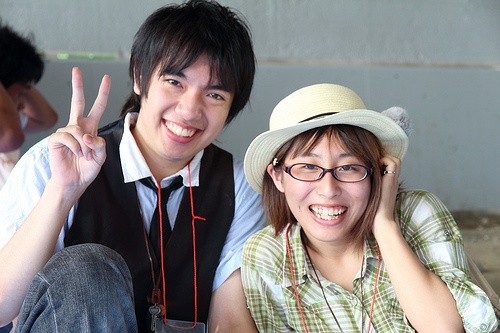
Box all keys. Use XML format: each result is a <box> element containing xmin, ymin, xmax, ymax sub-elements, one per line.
<box><xmin>149</xmin><ymin>303</ymin><xmax>161</xmax><ymax>331</ymax></box>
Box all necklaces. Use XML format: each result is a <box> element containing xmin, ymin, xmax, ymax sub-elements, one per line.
<box><xmin>287</xmin><ymin>223</ymin><xmax>381</xmax><ymax>333</ymax></box>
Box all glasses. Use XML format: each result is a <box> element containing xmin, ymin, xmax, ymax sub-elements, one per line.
<box><xmin>280</xmin><ymin>164</ymin><xmax>374</xmax><ymax>183</ymax></box>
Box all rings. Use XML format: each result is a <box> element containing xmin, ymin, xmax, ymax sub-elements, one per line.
<box><xmin>383</xmin><ymin>171</ymin><xmax>397</xmax><ymax>174</ymax></box>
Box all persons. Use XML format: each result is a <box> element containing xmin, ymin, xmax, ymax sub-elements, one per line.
<box><xmin>241</xmin><ymin>83</ymin><xmax>500</xmax><ymax>333</ymax></box>
<box><xmin>0</xmin><ymin>26</ymin><xmax>57</xmax><ymax>191</ymax></box>
<box><xmin>0</xmin><ymin>0</ymin><xmax>267</xmax><ymax>332</ymax></box>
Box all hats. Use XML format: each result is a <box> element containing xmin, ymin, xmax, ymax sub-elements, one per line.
<box><xmin>243</xmin><ymin>83</ymin><xmax>409</xmax><ymax>194</ymax></box>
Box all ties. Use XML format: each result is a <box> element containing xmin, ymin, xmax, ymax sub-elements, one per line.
<box><xmin>136</xmin><ymin>176</ymin><xmax>184</xmax><ymax>265</ymax></box>
